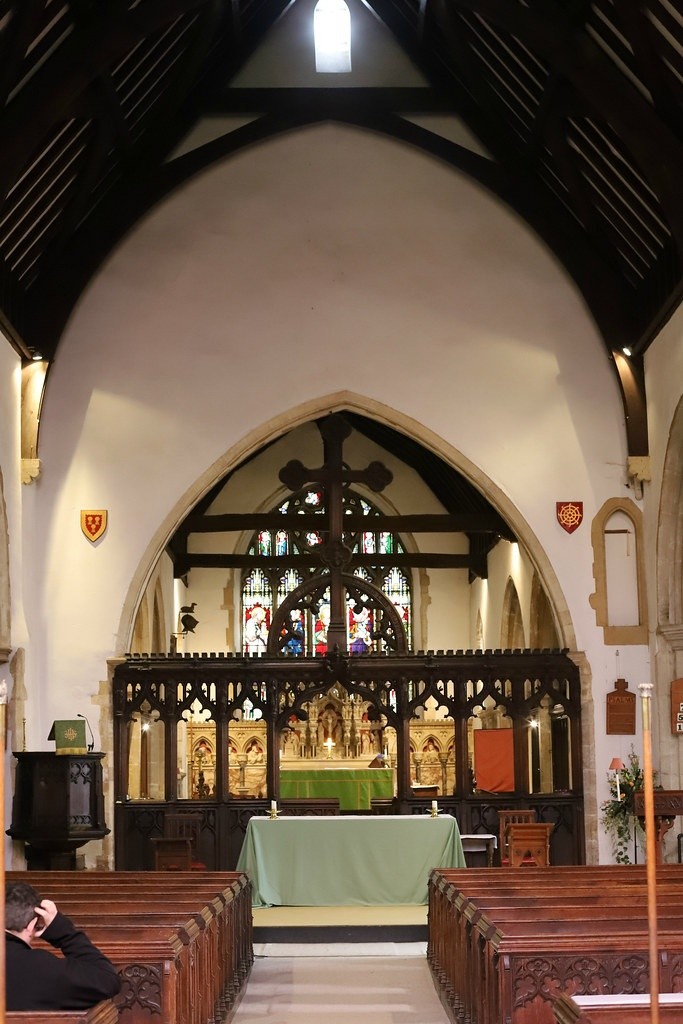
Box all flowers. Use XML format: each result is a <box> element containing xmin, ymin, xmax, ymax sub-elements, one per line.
<box><xmin>599</xmin><ymin>743</ymin><xmax>666</xmax><ymax>865</ymax></box>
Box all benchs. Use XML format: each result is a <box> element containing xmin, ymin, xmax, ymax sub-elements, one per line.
<box><xmin>427</xmin><ymin>865</ymin><xmax>683</xmax><ymax>1024</ymax></box>
<box><xmin>4</xmin><ymin>870</ymin><xmax>255</xmax><ymax>1024</ymax></box>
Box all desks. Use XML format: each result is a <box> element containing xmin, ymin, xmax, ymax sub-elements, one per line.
<box><xmin>235</xmin><ymin>814</ymin><xmax>467</xmax><ymax>908</ymax></box>
<box><xmin>279</xmin><ymin>768</ymin><xmax>394</xmax><ymax>810</ymax></box>
<box><xmin>460</xmin><ymin>834</ymin><xmax>497</xmax><ymax>867</ymax></box>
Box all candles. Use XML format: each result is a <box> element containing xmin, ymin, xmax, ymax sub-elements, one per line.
<box><xmin>432</xmin><ymin>801</ymin><xmax>437</xmax><ymax>807</ymax></box>
<box><xmin>271</xmin><ymin>800</ymin><xmax>276</xmax><ymax>809</ymax></box>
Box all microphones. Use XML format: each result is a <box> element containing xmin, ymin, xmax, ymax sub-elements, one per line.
<box><xmin>77</xmin><ymin>714</ymin><xmax>94</xmax><ymax>751</ymax></box>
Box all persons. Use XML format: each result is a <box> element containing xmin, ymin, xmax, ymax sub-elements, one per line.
<box><xmin>335</xmin><ymin>723</ymin><xmax>342</xmax><ymax>743</ymax></box>
<box><xmin>362</xmin><ymin>731</ymin><xmax>370</xmax><ymax>754</ymax></box>
<box><xmin>5</xmin><ymin>880</ymin><xmax>122</xmax><ymax>1011</ymax></box>
<box><xmin>318</xmin><ymin>723</ymin><xmax>324</xmax><ymax>746</ymax></box>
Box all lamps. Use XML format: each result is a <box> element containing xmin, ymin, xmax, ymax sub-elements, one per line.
<box><xmin>610</xmin><ymin>758</ymin><xmax>625</xmax><ymax>801</ymax></box>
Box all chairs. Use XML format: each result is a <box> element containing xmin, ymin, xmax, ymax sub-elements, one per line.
<box><xmin>496</xmin><ymin>810</ymin><xmax>538</xmax><ymax>867</ymax></box>
